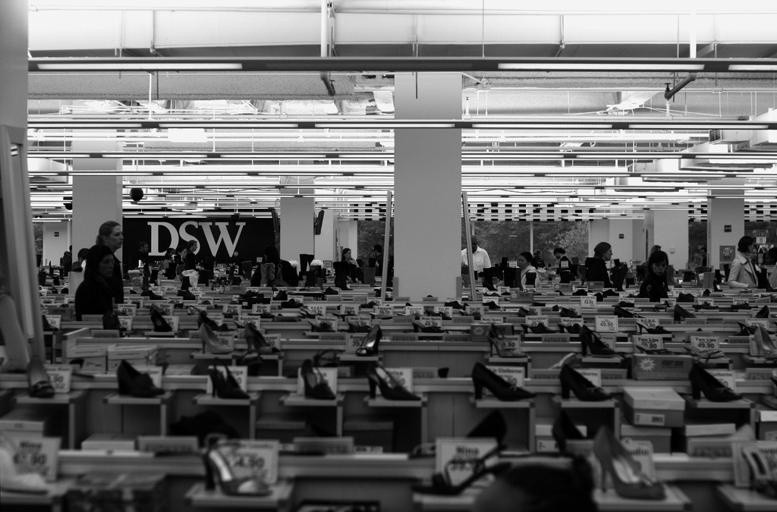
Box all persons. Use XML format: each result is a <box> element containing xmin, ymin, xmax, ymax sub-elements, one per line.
<box><xmin>135</xmin><ymin>234</ymin><xmax>396</xmax><ymax>291</ymax></box>
<box><xmin>582</xmin><ymin>241</ymin><xmax>674</xmax><ymax>303</ymax></box>
<box><xmin>728</xmin><ymin>232</ymin><xmax>776</xmax><ymax>294</ymax></box>
<box><xmin>74</xmin><ymin>221</ymin><xmax>126</xmax><ymax>330</ymax></box>
<box><xmin>71</xmin><ymin>247</ymin><xmax>90</xmax><ymax>271</ymax></box>
<box><xmin>459</xmin><ymin>233</ymin><xmax>579</xmax><ymax>289</ymax></box>
<box><xmin>62</xmin><ymin>245</ymin><xmax>72</xmax><ymax>277</ymax></box>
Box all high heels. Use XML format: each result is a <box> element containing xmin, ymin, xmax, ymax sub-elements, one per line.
<box><xmin>299</xmin><ymin>357</ymin><xmax>337</xmax><ymax>400</ymax></box>
<box><xmin>117</xmin><ymin>360</ymin><xmax>166</xmax><ymax>398</ymax></box>
<box><xmin>741</xmin><ymin>441</ymin><xmax>777</xmax><ymax>500</ymax></box>
<box><xmin>409</xmin><ymin>447</ymin><xmax>513</xmax><ymax>497</ymax></box>
<box><xmin>199</xmin><ymin>433</ymin><xmax>273</xmax><ymax>497</ymax></box>
<box><xmin>33</xmin><ymin>285</ymin><xmax>776</xmax><ymax>359</ymax></box>
<box><xmin>559</xmin><ymin>362</ymin><xmax>615</xmax><ymax>403</ymax></box>
<box><xmin>366</xmin><ymin>361</ymin><xmax>422</xmax><ymax>403</ymax></box>
<box><xmin>207</xmin><ymin>357</ymin><xmax>252</xmax><ymax>401</ymax></box>
<box><xmin>470</xmin><ymin>360</ymin><xmax>537</xmax><ymax>401</ymax></box>
<box><xmin>593</xmin><ymin>426</ymin><xmax>666</xmax><ymax>500</ymax></box>
<box><xmin>688</xmin><ymin>360</ymin><xmax>743</xmax><ymax>403</ymax></box>
<box><xmin>0</xmin><ymin>429</ymin><xmax>52</xmax><ymax>494</ymax></box>
<box><xmin>25</xmin><ymin>355</ymin><xmax>56</xmax><ymax>398</ymax></box>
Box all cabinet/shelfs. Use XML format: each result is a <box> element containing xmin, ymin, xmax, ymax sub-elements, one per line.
<box><xmin>0</xmin><ymin>257</ymin><xmax>777</xmax><ymax>512</ymax></box>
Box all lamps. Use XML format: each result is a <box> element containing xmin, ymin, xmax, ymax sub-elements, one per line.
<box><xmin>12</xmin><ymin>47</ymin><xmax>777</xmax><ymax>222</ymax></box>
